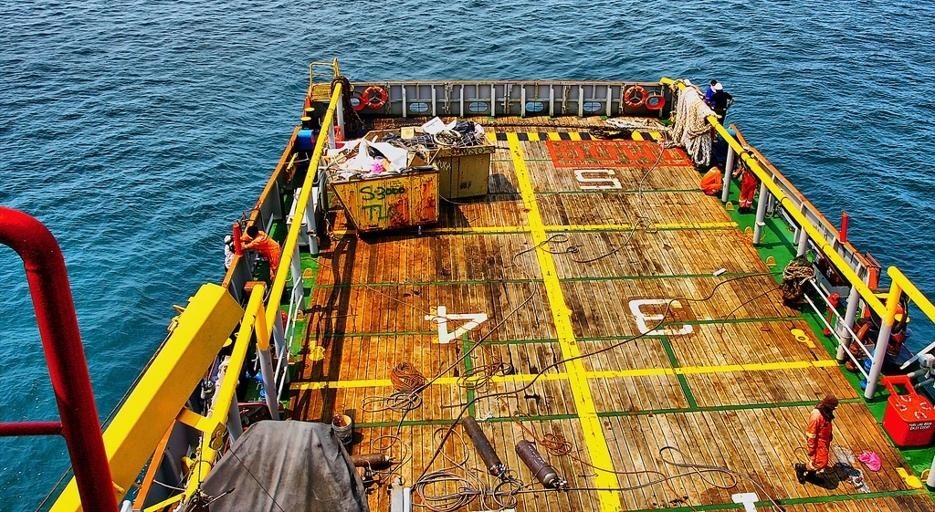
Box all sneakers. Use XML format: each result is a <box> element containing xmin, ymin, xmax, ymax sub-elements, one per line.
<box><xmin>794</xmin><ymin>463</ymin><xmax>816</xmax><ymax>485</ymax></box>
<box><xmin>738</xmin><ymin>207</ymin><xmax>751</xmax><ymax>213</ymax></box>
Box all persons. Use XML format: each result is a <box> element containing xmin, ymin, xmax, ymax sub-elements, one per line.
<box><xmin>707</xmin><ymin>82</ymin><xmax>735</xmax><ymax>128</ymax></box>
<box><xmin>737</xmin><ymin>165</ymin><xmax>760</xmax><ymax>213</ymax></box>
<box><xmin>223</xmin><ymin>234</ymin><xmax>236</xmax><ymax>273</ymax></box>
<box><xmin>704</xmin><ymin>80</ymin><xmax>718</xmax><ymax>110</ymax></box>
<box><xmin>238</xmin><ymin>225</ymin><xmax>283</xmax><ymax>279</ymax></box>
<box><xmin>699</xmin><ymin>161</ymin><xmax>725</xmax><ymax>196</ymax></box>
<box><xmin>794</xmin><ymin>394</ymin><xmax>838</xmax><ymax>484</ymax></box>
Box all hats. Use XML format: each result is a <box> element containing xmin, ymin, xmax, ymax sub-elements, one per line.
<box><xmin>713</xmin><ymin>83</ymin><xmax>723</xmax><ymax>90</ymax></box>
<box><xmin>823</xmin><ymin>394</ymin><xmax>838</xmax><ymax>409</ymax></box>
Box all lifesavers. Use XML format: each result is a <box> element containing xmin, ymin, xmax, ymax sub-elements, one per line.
<box><xmin>362</xmin><ymin>86</ymin><xmax>388</xmax><ymax>109</ymax></box>
<box><xmin>624</xmin><ymin>86</ymin><xmax>647</xmax><ymax>108</ymax></box>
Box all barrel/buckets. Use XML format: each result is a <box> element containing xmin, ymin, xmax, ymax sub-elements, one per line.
<box><xmin>331</xmin><ymin>415</ymin><xmax>353</xmax><ymax>445</ymax></box>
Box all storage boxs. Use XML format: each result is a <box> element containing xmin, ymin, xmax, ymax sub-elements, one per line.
<box><xmin>835</xmin><ymin>317</ymin><xmax>874</xmax><ymax>353</ymax></box>
<box><xmin>813</xmin><ymin>263</ymin><xmax>848</xmax><ymax>297</ymax></box>
<box><xmin>883</xmin><ymin>395</ymin><xmax>935</xmax><ymax>447</ymax></box>
<box><xmin>286</xmin><ymin>186</ymin><xmax>319</xmax><ymax>246</ymax></box>
